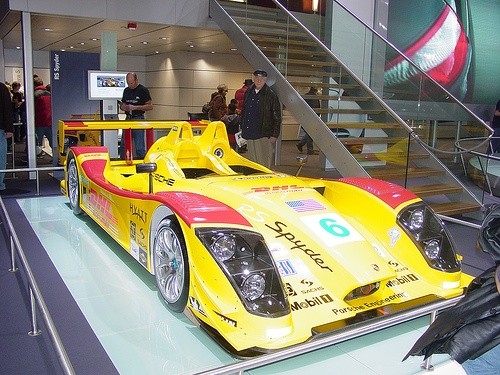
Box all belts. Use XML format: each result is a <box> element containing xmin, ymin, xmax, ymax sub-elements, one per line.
<box><xmin>127</xmin><ymin>115</ymin><xmax>142</xmax><ymax>118</ymax></box>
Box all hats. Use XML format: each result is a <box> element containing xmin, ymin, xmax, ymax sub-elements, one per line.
<box><xmin>217</xmin><ymin>83</ymin><xmax>228</xmax><ymax>90</ymax></box>
<box><xmin>243</xmin><ymin>79</ymin><xmax>254</xmax><ymax>84</ymax></box>
<box><xmin>254</xmin><ymin>71</ymin><xmax>267</xmax><ymax>77</ymax></box>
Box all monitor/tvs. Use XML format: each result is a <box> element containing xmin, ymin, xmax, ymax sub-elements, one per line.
<box><xmin>88</xmin><ymin>69</ymin><xmax>129</xmax><ymax>100</ymax></box>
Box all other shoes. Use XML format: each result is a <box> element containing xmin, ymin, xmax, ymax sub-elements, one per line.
<box><xmin>0</xmin><ymin>187</ymin><xmax>11</xmax><ymax>195</ymax></box>
<box><xmin>296</xmin><ymin>143</ymin><xmax>302</xmax><ymax>151</ymax></box>
<box><xmin>307</xmin><ymin>150</ymin><xmax>319</xmax><ymax>155</ymax></box>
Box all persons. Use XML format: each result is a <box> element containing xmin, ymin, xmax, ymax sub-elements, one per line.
<box><xmin>0</xmin><ymin>81</ymin><xmax>15</xmax><ymax>191</ymax></box>
<box><xmin>487</xmin><ymin>99</ymin><xmax>500</xmax><ymax>155</ymax></box>
<box><xmin>6</xmin><ymin>73</ymin><xmax>54</xmax><ymax>164</ymax></box>
<box><xmin>207</xmin><ymin>79</ymin><xmax>254</xmax><ymax>155</ymax></box>
<box><xmin>120</xmin><ymin>72</ymin><xmax>153</xmax><ymax>161</ymax></box>
<box><xmin>240</xmin><ymin>70</ymin><xmax>281</xmax><ymax>172</ymax></box>
<box><xmin>296</xmin><ymin>87</ymin><xmax>320</xmax><ymax>155</ymax></box>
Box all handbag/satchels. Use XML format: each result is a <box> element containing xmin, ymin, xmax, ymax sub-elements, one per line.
<box><xmin>202</xmin><ymin>104</ymin><xmax>210</xmax><ymax>114</ymax></box>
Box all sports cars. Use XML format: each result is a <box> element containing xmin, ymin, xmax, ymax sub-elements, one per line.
<box><xmin>102</xmin><ymin>79</ymin><xmax>120</xmax><ymax>87</ymax></box>
<box><xmin>57</xmin><ymin>119</ymin><xmax>476</xmax><ymax>357</ymax></box>
